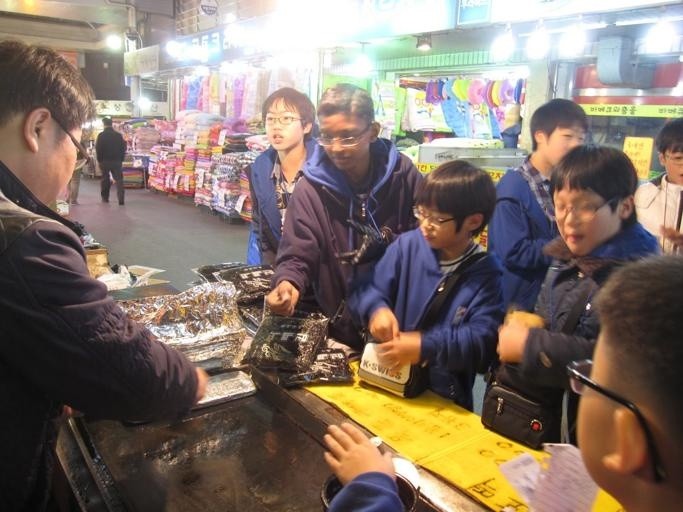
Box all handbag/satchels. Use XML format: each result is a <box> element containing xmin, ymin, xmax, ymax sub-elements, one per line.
<box><xmin>111</xmin><ymin>132</ymin><xmax>125</xmax><ymax>162</ymax></box>
<box><xmin>480</xmin><ymin>269</ymin><xmax>611</xmax><ymax>451</ymax></box>
<box><xmin>357</xmin><ymin>251</ymin><xmax>487</xmax><ymax>400</ymax></box>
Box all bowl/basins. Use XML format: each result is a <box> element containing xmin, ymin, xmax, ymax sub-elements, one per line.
<box><xmin>320</xmin><ymin>471</ymin><xmax>418</xmax><ymax>512</ymax></box>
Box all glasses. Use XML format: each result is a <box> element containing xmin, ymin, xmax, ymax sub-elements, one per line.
<box><xmin>663</xmin><ymin>152</ymin><xmax>683</xmax><ymax>166</ymax></box>
<box><xmin>312</xmin><ymin>122</ymin><xmax>374</xmax><ymax>148</ymax></box>
<box><xmin>49</xmin><ymin>113</ymin><xmax>90</xmax><ymax>172</ymax></box>
<box><xmin>263</xmin><ymin>113</ymin><xmax>310</xmax><ymax>126</ymax></box>
<box><xmin>413</xmin><ymin>202</ymin><xmax>465</xmax><ymax>226</ymax></box>
<box><xmin>543</xmin><ymin>194</ymin><xmax>619</xmax><ymax>222</ymax></box>
<box><xmin>565</xmin><ymin>358</ymin><xmax>669</xmax><ymax>485</ymax></box>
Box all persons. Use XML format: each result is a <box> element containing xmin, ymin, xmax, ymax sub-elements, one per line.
<box><xmin>634</xmin><ymin>118</ymin><xmax>683</xmax><ymax>257</ymax></box>
<box><xmin>266</xmin><ymin>82</ymin><xmax>427</xmax><ymax>350</ymax></box>
<box><xmin>322</xmin><ymin>249</ymin><xmax>682</xmax><ymax>511</ymax></box>
<box><xmin>0</xmin><ymin>40</ymin><xmax>207</xmax><ymax>511</ymax></box>
<box><xmin>346</xmin><ymin>164</ymin><xmax>507</xmax><ymax>412</ymax></box>
<box><xmin>488</xmin><ymin>99</ymin><xmax>591</xmax><ymax>311</ymax></box>
<box><xmin>496</xmin><ymin>143</ymin><xmax>664</xmax><ymax>444</ymax></box>
<box><xmin>94</xmin><ymin>116</ymin><xmax>128</xmax><ymax>207</ymax></box>
<box><xmin>244</xmin><ymin>87</ymin><xmax>323</xmax><ymax>254</ymax></box>
<box><xmin>65</xmin><ymin>142</ymin><xmax>86</xmax><ymax>206</ymax></box>
